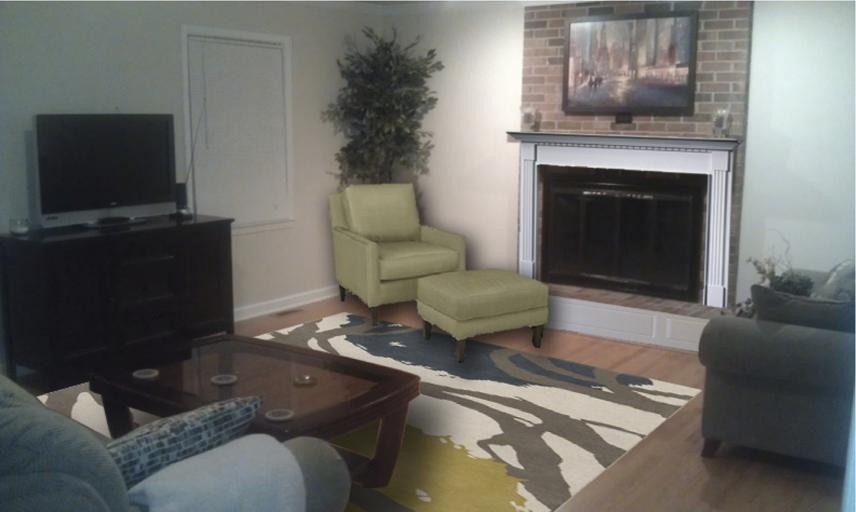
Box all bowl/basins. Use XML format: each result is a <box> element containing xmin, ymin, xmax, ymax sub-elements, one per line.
<box><xmin>8</xmin><ymin>217</ymin><xmax>30</xmax><ymax>235</ymax></box>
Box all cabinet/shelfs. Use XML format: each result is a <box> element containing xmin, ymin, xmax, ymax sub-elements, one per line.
<box><xmin>1</xmin><ymin>213</ymin><xmax>235</xmax><ymax>381</ymax></box>
<box><xmin>507</xmin><ymin>127</ymin><xmax>741</xmax><ymax>310</ymax></box>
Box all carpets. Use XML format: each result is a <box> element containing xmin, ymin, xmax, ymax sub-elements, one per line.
<box><xmin>36</xmin><ymin>310</ymin><xmax>704</xmax><ymax>512</ymax></box>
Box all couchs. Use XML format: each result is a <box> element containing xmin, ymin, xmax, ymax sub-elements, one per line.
<box><xmin>416</xmin><ymin>268</ymin><xmax>550</xmax><ymax>363</ymax></box>
<box><xmin>0</xmin><ymin>375</ymin><xmax>353</xmax><ymax>511</ymax></box>
<box><xmin>699</xmin><ymin>257</ymin><xmax>855</xmax><ymax>468</ymax></box>
<box><xmin>329</xmin><ymin>182</ymin><xmax>468</xmax><ymax>326</ymax></box>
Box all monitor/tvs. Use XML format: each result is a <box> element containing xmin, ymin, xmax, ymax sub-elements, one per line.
<box><xmin>34</xmin><ymin>113</ymin><xmax>176</xmax><ymax>226</ymax></box>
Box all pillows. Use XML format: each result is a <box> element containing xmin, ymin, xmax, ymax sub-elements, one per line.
<box><xmin>107</xmin><ymin>392</ymin><xmax>265</xmax><ymax>490</ymax></box>
<box><xmin>751</xmin><ymin>283</ymin><xmax>855</xmax><ymax>332</ymax></box>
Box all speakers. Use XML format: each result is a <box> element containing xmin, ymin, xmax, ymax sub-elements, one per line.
<box><xmin>176</xmin><ymin>183</ymin><xmax>187</xmax><ymax>210</ymax></box>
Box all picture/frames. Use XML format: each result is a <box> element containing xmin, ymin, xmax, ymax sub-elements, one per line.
<box><xmin>559</xmin><ymin>8</ymin><xmax>699</xmax><ymax>117</ymax></box>
